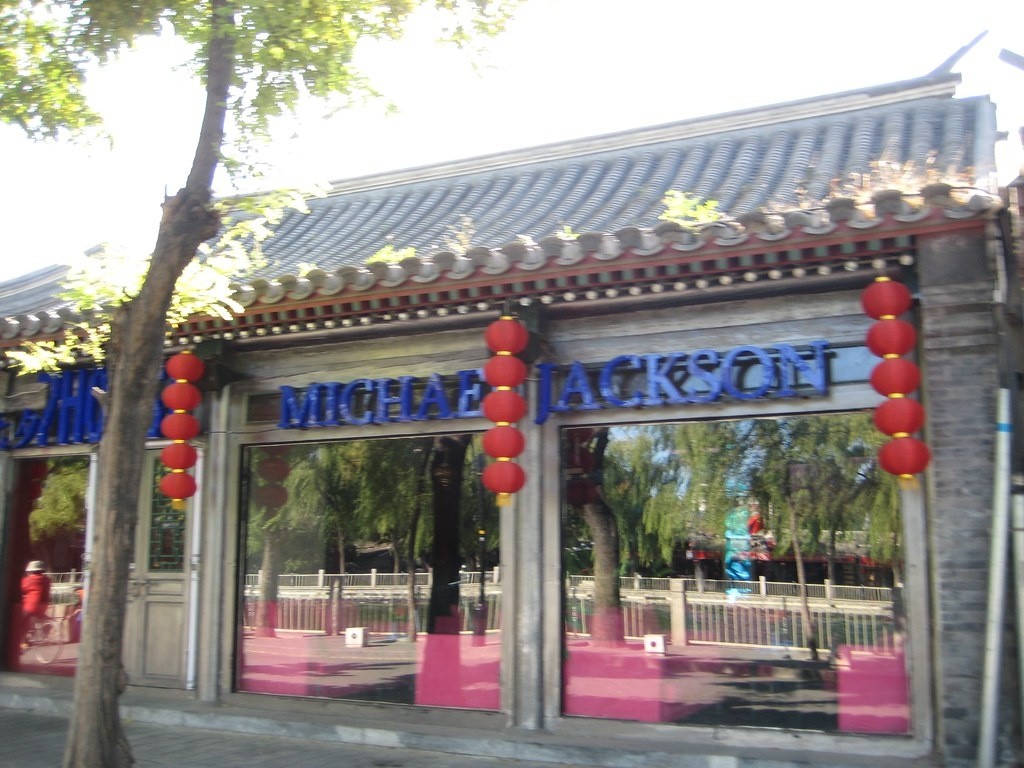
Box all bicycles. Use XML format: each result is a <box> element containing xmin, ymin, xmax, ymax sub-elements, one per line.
<box><xmin>20</xmin><ymin>616</ymin><xmax>65</xmax><ymax>665</ymax></box>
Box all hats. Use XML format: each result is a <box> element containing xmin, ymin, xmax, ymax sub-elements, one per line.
<box><xmin>24</xmin><ymin>560</ymin><xmax>46</xmax><ymax>571</ymax></box>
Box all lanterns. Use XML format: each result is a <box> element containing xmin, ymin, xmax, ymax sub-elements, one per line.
<box><xmin>160</xmin><ymin>350</ymin><xmax>204</xmax><ymax>509</ymax></box>
<box><xmin>482</xmin><ymin>316</ymin><xmax>529</xmax><ymax>506</ymax></box>
<box><xmin>861</xmin><ymin>277</ymin><xmax>931</xmax><ymax>491</ymax></box>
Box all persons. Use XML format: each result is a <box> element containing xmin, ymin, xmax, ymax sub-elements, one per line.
<box><xmin>20</xmin><ymin>561</ymin><xmax>50</xmax><ymax>651</ymax></box>
